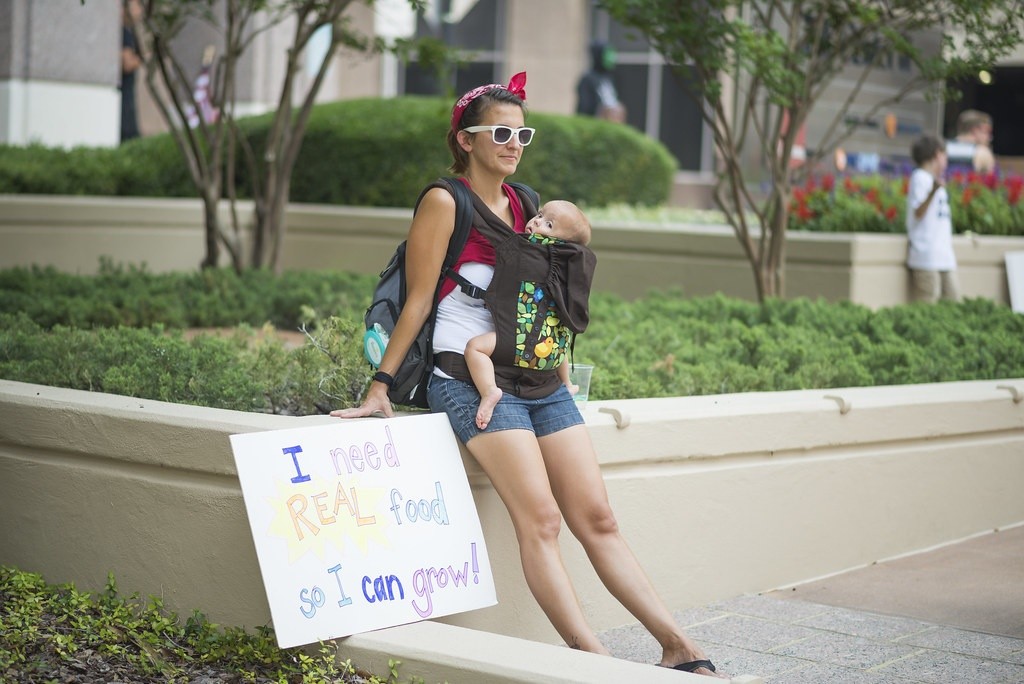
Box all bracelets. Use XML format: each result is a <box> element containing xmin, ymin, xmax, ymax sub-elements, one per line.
<box><xmin>371</xmin><ymin>371</ymin><xmax>395</xmax><ymax>387</ymax></box>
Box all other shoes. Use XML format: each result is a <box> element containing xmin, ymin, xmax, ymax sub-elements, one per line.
<box><xmin>656</xmin><ymin>659</ymin><xmax>714</xmax><ymax>672</ymax></box>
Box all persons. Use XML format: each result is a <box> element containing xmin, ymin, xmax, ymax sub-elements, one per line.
<box><xmin>329</xmin><ymin>84</ymin><xmax>728</xmax><ymax>680</ymax></box>
<box><xmin>463</xmin><ymin>200</ymin><xmax>593</xmax><ymax>429</ymax></box>
<box><xmin>903</xmin><ymin>108</ymin><xmax>999</xmax><ymax>303</ymax></box>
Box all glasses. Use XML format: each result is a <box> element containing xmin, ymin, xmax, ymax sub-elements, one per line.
<box><xmin>463</xmin><ymin>126</ymin><xmax>535</xmax><ymax>147</ymax></box>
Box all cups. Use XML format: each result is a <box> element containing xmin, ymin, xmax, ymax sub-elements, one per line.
<box><xmin>567</xmin><ymin>364</ymin><xmax>594</xmax><ymax>403</ymax></box>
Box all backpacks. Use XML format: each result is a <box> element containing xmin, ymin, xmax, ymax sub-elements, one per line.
<box><xmin>364</xmin><ymin>176</ymin><xmax>542</xmax><ymax>409</ymax></box>
